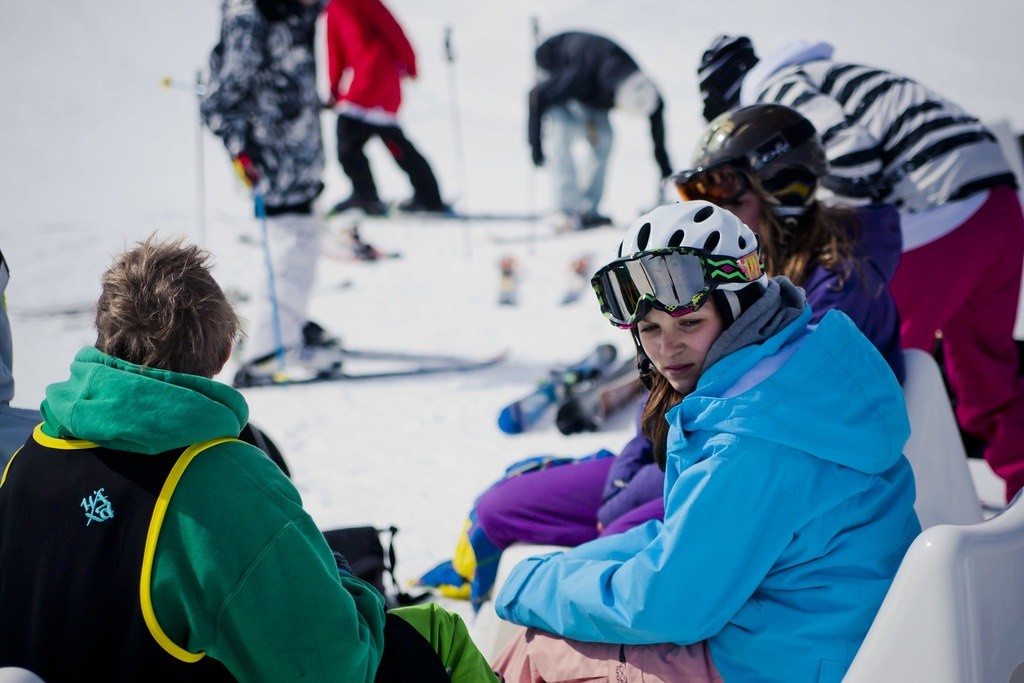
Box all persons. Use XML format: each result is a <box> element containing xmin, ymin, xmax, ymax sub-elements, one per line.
<box><xmin>0</xmin><ymin>245</ymin><xmax>506</xmax><ymax>683</ymax></box>
<box><xmin>198</xmin><ymin>0</ymin><xmax>345</xmax><ymax>385</ymax></box>
<box><xmin>0</xmin><ymin>248</ymin><xmax>16</xmax><ymax>407</ymax></box>
<box><xmin>315</xmin><ymin>0</ymin><xmax>451</xmax><ymax>219</ymax></box>
<box><xmin>528</xmin><ymin>30</ymin><xmax>674</xmax><ymax>233</ymax></box>
<box><xmin>699</xmin><ymin>34</ymin><xmax>1024</xmax><ymax>501</ymax></box>
<box><xmin>452</xmin><ymin>104</ymin><xmax>904</xmax><ymax>619</ymax></box>
<box><xmin>497</xmin><ymin>198</ymin><xmax>925</xmax><ymax>683</ymax></box>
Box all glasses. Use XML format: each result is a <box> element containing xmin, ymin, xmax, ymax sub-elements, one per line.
<box><xmin>675</xmin><ymin>156</ymin><xmax>751</xmax><ymax>206</ymax></box>
<box><xmin>592</xmin><ymin>247</ymin><xmax>709</xmax><ymax>329</ymax></box>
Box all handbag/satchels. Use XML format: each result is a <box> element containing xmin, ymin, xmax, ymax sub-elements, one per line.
<box><xmin>320</xmin><ymin>526</ymin><xmax>398</xmax><ymax>596</ymax></box>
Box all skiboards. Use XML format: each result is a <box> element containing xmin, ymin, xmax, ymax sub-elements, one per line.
<box><xmin>229</xmin><ymin>339</ymin><xmax>513</xmax><ymax>388</ymax></box>
<box><xmin>497</xmin><ymin>344</ymin><xmax>643</xmax><ymax>435</ymax></box>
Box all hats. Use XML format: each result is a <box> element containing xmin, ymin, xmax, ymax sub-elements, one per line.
<box><xmin>617</xmin><ymin>74</ymin><xmax>659</xmax><ymax>114</ymax></box>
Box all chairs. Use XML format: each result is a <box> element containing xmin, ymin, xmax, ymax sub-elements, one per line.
<box><xmin>840</xmin><ymin>488</ymin><xmax>1024</xmax><ymax>683</ymax></box>
<box><xmin>894</xmin><ymin>349</ymin><xmax>984</xmax><ymax>529</ymax></box>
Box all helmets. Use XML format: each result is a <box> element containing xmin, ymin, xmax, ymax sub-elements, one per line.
<box><xmin>677</xmin><ymin>103</ymin><xmax>826</xmax><ymax>235</ymax></box>
<box><xmin>592</xmin><ymin>200</ymin><xmax>769</xmax><ymax>292</ymax></box>
<box><xmin>696</xmin><ymin>35</ymin><xmax>760</xmax><ymax>121</ymax></box>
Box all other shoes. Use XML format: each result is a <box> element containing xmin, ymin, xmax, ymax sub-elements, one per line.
<box><xmin>398</xmin><ymin>192</ymin><xmax>448</xmax><ymax>214</ymax></box>
<box><xmin>581</xmin><ymin>209</ymin><xmax>612</xmax><ymax>229</ymax></box>
<box><xmin>240</xmin><ymin>337</ymin><xmax>343</xmax><ymax>383</ymax></box>
<box><xmin>329</xmin><ymin>190</ymin><xmax>387</xmax><ymax>217</ymax></box>
